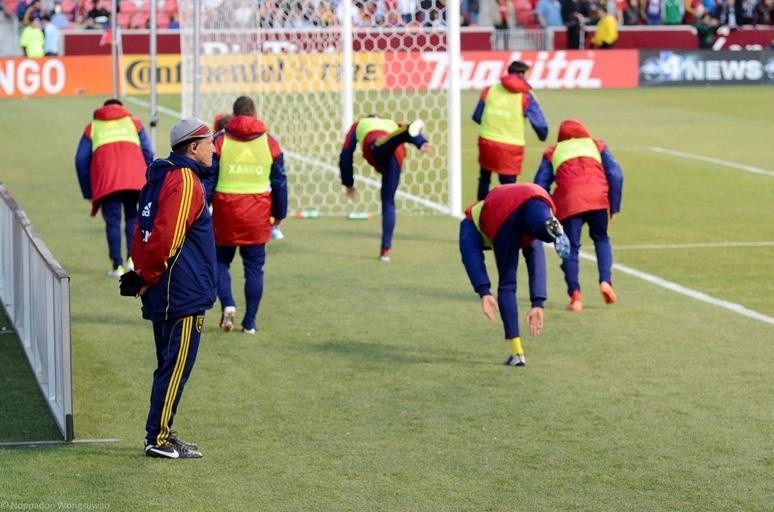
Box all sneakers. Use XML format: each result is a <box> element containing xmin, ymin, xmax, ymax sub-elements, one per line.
<box><xmin>543</xmin><ymin>216</ymin><xmax>571</xmax><ymax>259</ymax></box>
<box><xmin>238</xmin><ymin>320</ymin><xmax>257</xmax><ymax>335</ymax></box>
<box><xmin>107</xmin><ymin>264</ymin><xmax>126</xmax><ymax>277</ymax></box>
<box><xmin>565</xmin><ymin>300</ymin><xmax>583</xmax><ymax>313</ymax></box>
<box><xmin>502</xmin><ymin>352</ymin><xmax>526</xmax><ymax>366</ymax></box>
<box><xmin>408</xmin><ymin>118</ymin><xmax>425</xmax><ymax>138</ymax></box>
<box><xmin>144</xmin><ymin>439</ymin><xmax>204</xmax><ymax>459</ymax></box>
<box><xmin>381</xmin><ymin>246</ymin><xmax>390</xmax><ymax>261</ymax></box>
<box><xmin>166</xmin><ymin>428</ymin><xmax>198</xmax><ymax>450</ymax></box>
<box><xmin>219</xmin><ymin>297</ymin><xmax>237</xmax><ymax>333</ymax></box>
<box><xmin>124</xmin><ymin>256</ymin><xmax>134</xmax><ymax>271</ymax></box>
<box><xmin>599</xmin><ymin>280</ymin><xmax>616</xmax><ymax>304</ymax></box>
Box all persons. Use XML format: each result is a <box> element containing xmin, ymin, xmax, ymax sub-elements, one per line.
<box><xmin>459</xmin><ymin>180</ymin><xmax>571</xmax><ymax>369</ymax></box>
<box><xmin>201</xmin><ymin>95</ymin><xmax>289</xmax><ymax>334</ymax></box>
<box><xmin>165</xmin><ymin>0</ymin><xmax>446</xmax><ymax>31</ymax></box>
<box><xmin>567</xmin><ymin>6</ymin><xmax>620</xmax><ymax>47</ymax></box>
<box><xmin>471</xmin><ymin>60</ymin><xmax>550</xmax><ymax>205</ymax></box>
<box><xmin>339</xmin><ymin>112</ymin><xmax>432</xmax><ymax>262</ymax></box>
<box><xmin>698</xmin><ymin>14</ymin><xmax>730</xmax><ymax>51</ymax></box>
<box><xmin>531</xmin><ymin>120</ymin><xmax>625</xmax><ymax>312</ymax></box>
<box><xmin>74</xmin><ymin>98</ymin><xmax>156</xmax><ymax>276</ymax></box>
<box><xmin>460</xmin><ymin>0</ymin><xmax>773</xmax><ymax>27</ymax></box>
<box><xmin>118</xmin><ymin>117</ymin><xmax>219</xmax><ymax>461</ymax></box>
<box><xmin>0</xmin><ymin>0</ymin><xmax>114</xmax><ymax>59</ymax></box>
<box><xmin>213</xmin><ymin>112</ymin><xmax>283</xmax><ymax>241</ymax></box>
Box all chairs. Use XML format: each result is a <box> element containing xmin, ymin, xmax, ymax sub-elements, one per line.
<box><xmin>59</xmin><ymin>0</ymin><xmax>182</xmax><ymax>31</ymax></box>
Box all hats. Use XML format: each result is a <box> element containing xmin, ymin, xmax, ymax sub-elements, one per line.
<box><xmin>170</xmin><ymin>115</ymin><xmax>214</xmax><ymax>148</ymax></box>
